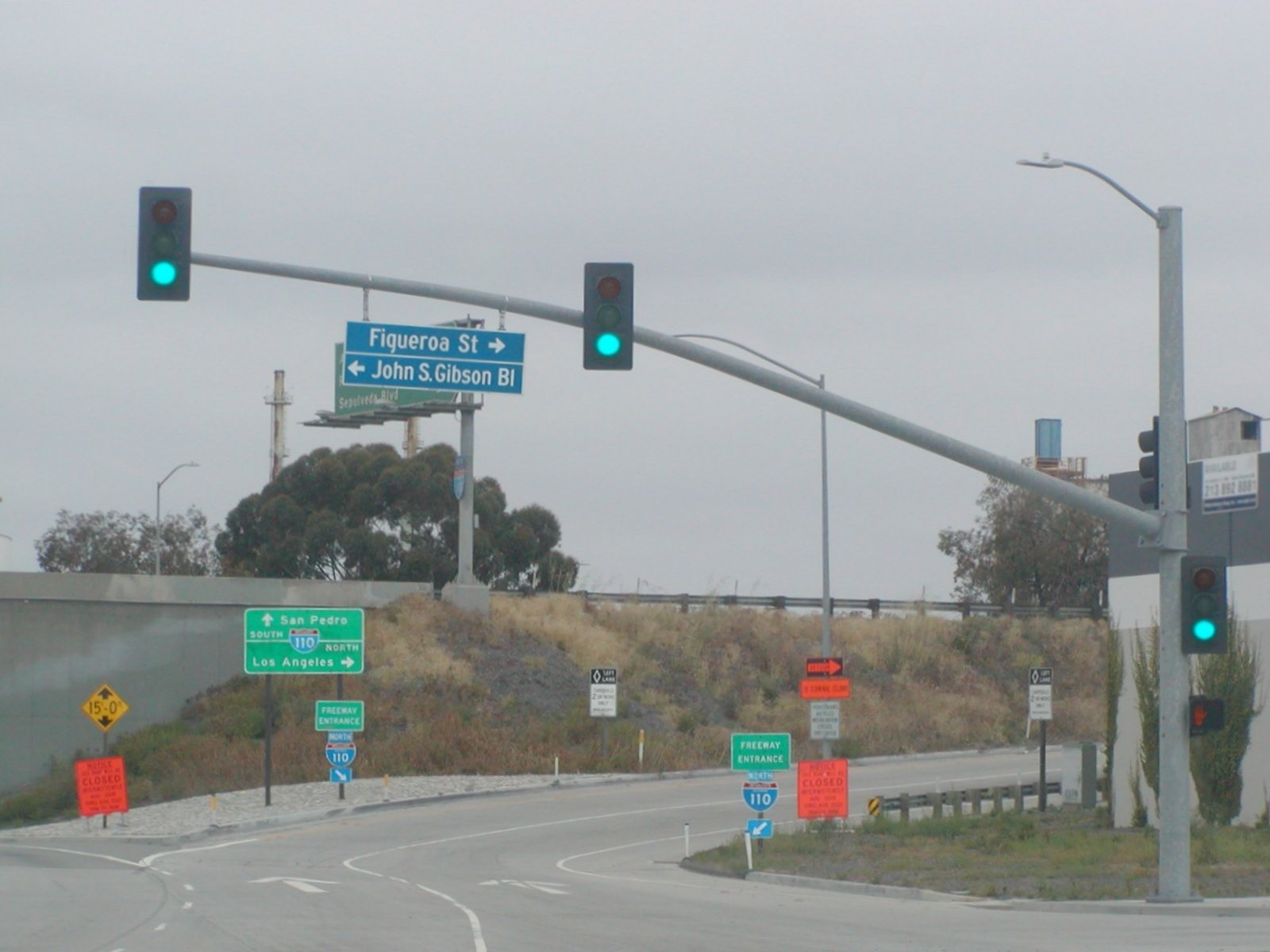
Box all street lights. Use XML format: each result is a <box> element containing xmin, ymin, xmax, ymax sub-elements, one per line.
<box><xmin>667</xmin><ymin>331</ymin><xmax>833</xmax><ymax>842</ymax></box>
<box><xmin>156</xmin><ymin>462</ymin><xmax>199</xmax><ymax>576</ymax></box>
<box><xmin>1015</xmin><ymin>154</ymin><xmax>1194</xmax><ymax>904</ymax></box>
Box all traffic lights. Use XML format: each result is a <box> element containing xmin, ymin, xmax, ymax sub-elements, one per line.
<box><xmin>581</xmin><ymin>261</ymin><xmax>637</xmax><ymax>373</ymax></box>
<box><xmin>1138</xmin><ymin>415</ymin><xmax>1189</xmax><ymax>511</ymax></box>
<box><xmin>135</xmin><ymin>186</ymin><xmax>192</xmax><ymax>301</ymax></box>
<box><xmin>1183</xmin><ymin>554</ymin><xmax>1226</xmax><ymax>657</ymax></box>
<box><xmin>1189</xmin><ymin>695</ymin><xmax>1225</xmax><ymax>737</ymax></box>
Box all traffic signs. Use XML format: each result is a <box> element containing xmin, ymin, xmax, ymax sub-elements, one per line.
<box><xmin>807</xmin><ymin>657</ymin><xmax>843</xmax><ymax>678</ymax></box>
<box><xmin>341</xmin><ymin>320</ymin><xmax>526</xmax><ymax>398</ymax></box>
<box><xmin>244</xmin><ymin>607</ymin><xmax>365</xmax><ymax>675</ymax></box>
